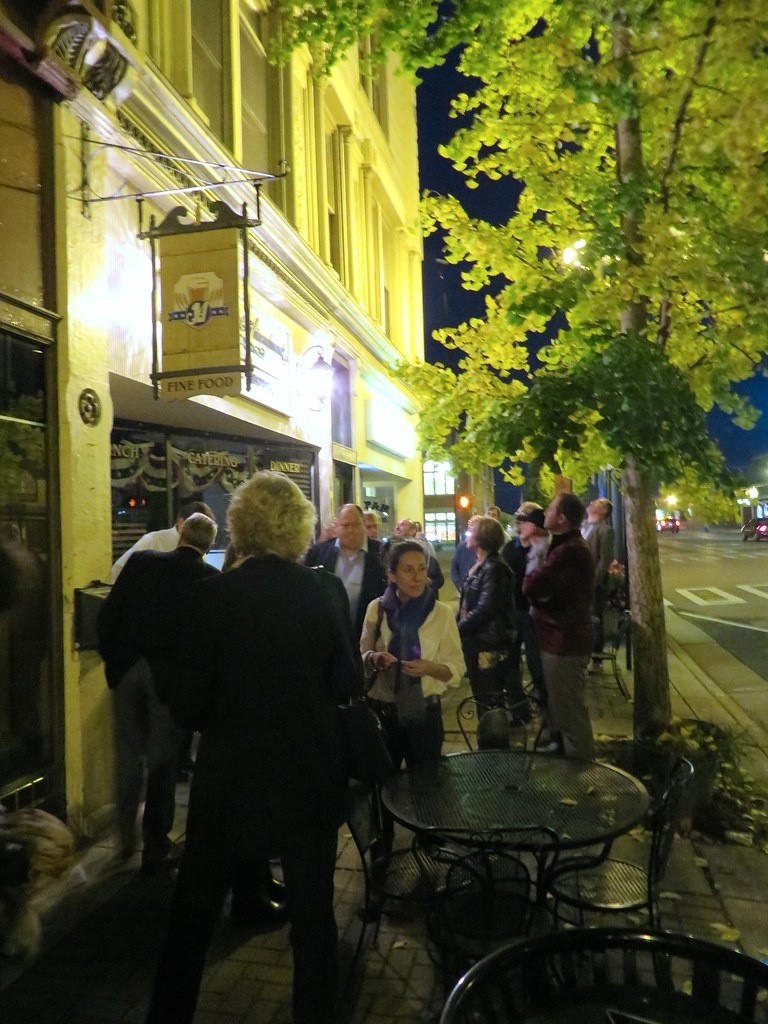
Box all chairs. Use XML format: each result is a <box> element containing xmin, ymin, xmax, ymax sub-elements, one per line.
<box><xmin>583</xmin><ymin>609</ymin><xmax>631</xmax><ymax>702</ymax></box>
<box><xmin>341</xmin><ymin>770</ymin><xmax>476</xmax><ymax>961</ymax></box>
<box><xmin>539</xmin><ymin>751</ymin><xmax>697</xmax><ymax>929</ymax></box>
<box><xmin>410</xmin><ymin>823</ymin><xmax>562</xmax><ymax>977</ymax></box>
<box><xmin>458</xmin><ymin>689</ymin><xmax>551</xmax><ymax>750</ymax></box>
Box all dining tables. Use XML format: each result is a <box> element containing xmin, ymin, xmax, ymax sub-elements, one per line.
<box><xmin>376</xmin><ymin>747</ymin><xmax>649</xmax><ymax>907</ymax></box>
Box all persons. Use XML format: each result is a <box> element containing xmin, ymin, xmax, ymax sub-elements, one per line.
<box><xmin>112</xmin><ymin>502</ymin><xmax>217</xmax><ymax>583</ymax></box>
<box><xmin>301</xmin><ymin>503</ymin><xmax>389</xmax><ymax>638</ymax></box>
<box><xmin>0</xmin><ymin>525</ymin><xmax>52</xmax><ymax>770</ymax></box>
<box><xmin>450</xmin><ymin>515</ymin><xmax>482</xmax><ymax>593</ymax></box>
<box><xmin>580</xmin><ymin>498</ymin><xmax>615</xmax><ymax>673</ymax></box>
<box><xmin>97</xmin><ymin>513</ymin><xmax>224</xmax><ymax>875</ymax></box>
<box><xmin>409</xmin><ymin>521</ymin><xmax>437</xmax><ymax>559</ymax></box>
<box><xmin>395</xmin><ymin>519</ymin><xmax>417</xmax><ymax>539</ymax></box>
<box><xmin>482</xmin><ymin>506</ymin><xmax>511</xmax><ymax>553</ymax></box>
<box><xmin>145</xmin><ymin>472</ymin><xmax>360</xmax><ymax>1024</ymax></box>
<box><xmin>521</xmin><ymin>492</ymin><xmax>597</xmax><ymax>786</ymax></box>
<box><xmin>516</xmin><ymin>508</ymin><xmax>559</xmax><ymax>752</ymax></box>
<box><xmin>359</xmin><ymin>540</ymin><xmax>466</xmax><ymax>872</ymax></box>
<box><xmin>457</xmin><ymin>515</ymin><xmax>518</xmax><ymax>793</ymax></box>
<box><xmin>501</xmin><ymin>502</ymin><xmax>543</xmax><ymax>730</ymax></box>
<box><xmin>318</xmin><ymin>510</ymin><xmax>388</xmax><ymax>551</ymax></box>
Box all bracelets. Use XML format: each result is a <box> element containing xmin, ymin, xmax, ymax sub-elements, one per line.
<box><xmin>369</xmin><ymin>652</ymin><xmax>377</xmax><ymax>672</ymax></box>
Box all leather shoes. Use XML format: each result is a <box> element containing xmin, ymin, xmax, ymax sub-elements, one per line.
<box><xmin>260</xmin><ymin>875</ymin><xmax>289</xmax><ymax>896</ymax></box>
<box><xmin>232</xmin><ymin>891</ymin><xmax>292</xmax><ymax>922</ymax></box>
<box><xmin>139</xmin><ymin>840</ymin><xmax>187</xmax><ymax>868</ymax></box>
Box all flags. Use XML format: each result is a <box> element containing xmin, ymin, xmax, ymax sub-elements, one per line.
<box><xmin>157</xmin><ymin>227</ymin><xmax>243</xmax><ymax>401</ymax></box>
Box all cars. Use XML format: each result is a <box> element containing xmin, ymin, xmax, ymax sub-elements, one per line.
<box><xmin>657</xmin><ymin>518</ymin><xmax>680</xmax><ymax>533</ymax></box>
<box><xmin>740</xmin><ymin>518</ymin><xmax>768</xmax><ymax>541</ymax></box>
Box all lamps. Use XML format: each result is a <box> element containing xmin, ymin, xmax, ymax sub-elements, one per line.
<box><xmin>302</xmin><ymin>344</ymin><xmax>336</xmax><ymax>371</ymax></box>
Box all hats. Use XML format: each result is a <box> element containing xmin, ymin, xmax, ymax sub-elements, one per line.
<box><xmin>517</xmin><ymin>509</ymin><xmax>549</xmax><ymax>529</ymax></box>
